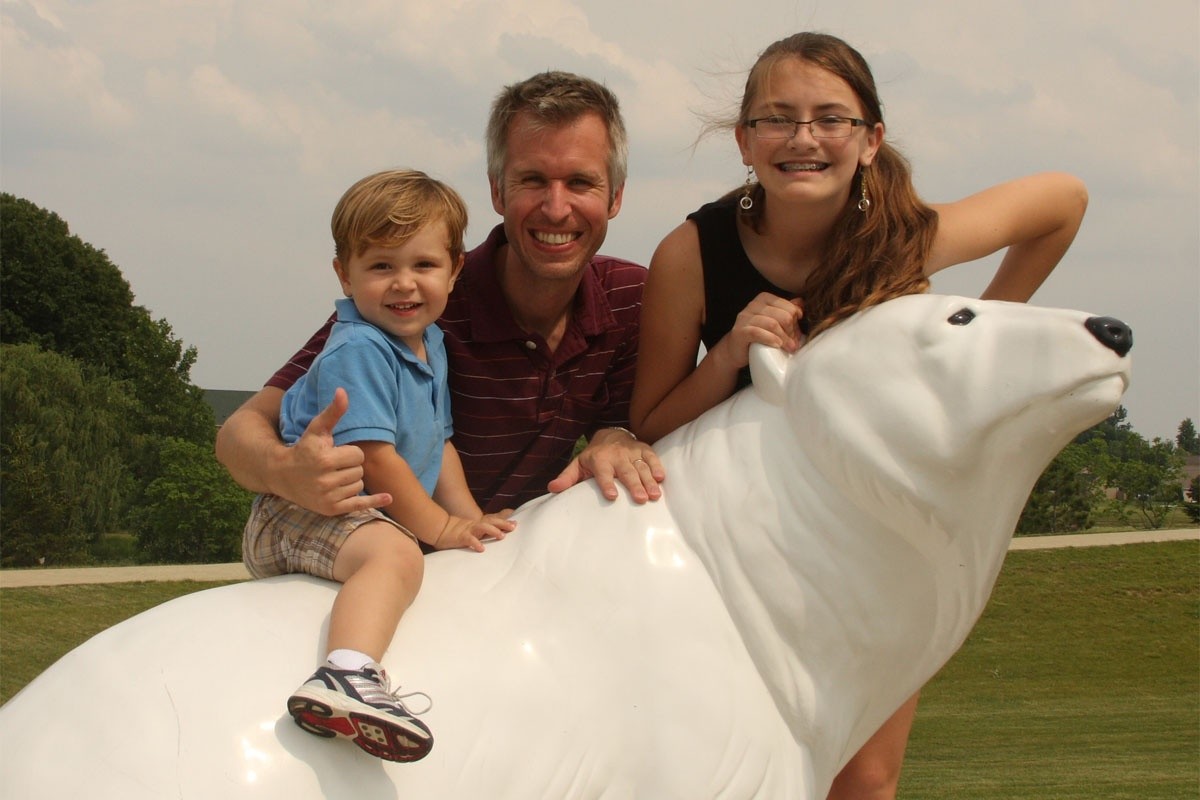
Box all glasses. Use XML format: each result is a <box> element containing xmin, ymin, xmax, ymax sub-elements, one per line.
<box><xmin>745</xmin><ymin>111</ymin><xmax>871</xmax><ymax>140</ymax></box>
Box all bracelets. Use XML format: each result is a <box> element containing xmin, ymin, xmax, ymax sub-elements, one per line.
<box><xmin>598</xmin><ymin>426</ymin><xmax>637</xmax><ymax>441</ymax></box>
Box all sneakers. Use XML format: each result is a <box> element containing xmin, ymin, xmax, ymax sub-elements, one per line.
<box><xmin>287</xmin><ymin>659</ymin><xmax>434</xmax><ymax>762</ymax></box>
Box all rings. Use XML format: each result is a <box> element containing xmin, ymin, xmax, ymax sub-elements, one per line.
<box><xmin>632</xmin><ymin>458</ymin><xmax>644</xmax><ymax>463</ymax></box>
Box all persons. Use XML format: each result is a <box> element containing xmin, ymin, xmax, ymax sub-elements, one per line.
<box><xmin>634</xmin><ymin>32</ymin><xmax>1089</xmax><ymax>800</ymax></box>
<box><xmin>244</xmin><ymin>168</ymin><xmax>517</xmax><ymax>764</ymax></box>
<box><xmin>214</xmin><ymin>71</ymin><xmax>669</xmax><ymax>516</ymax></box>
